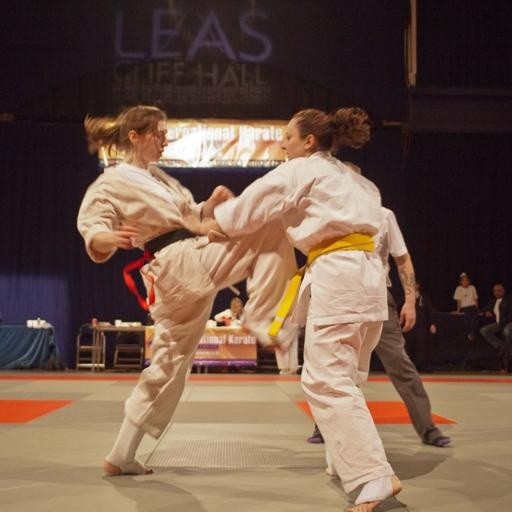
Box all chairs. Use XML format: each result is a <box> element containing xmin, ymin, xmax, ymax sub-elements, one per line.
<box><xmin>75</xmin><ymin>323</ymin><xmax>107</xmax><ymax>372</ymax></box>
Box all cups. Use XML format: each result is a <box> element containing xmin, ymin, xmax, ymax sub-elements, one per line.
<box><xmin>114</xmin><ymin>319</ymin><xmax>123</xmax><ymax>328</ymax></box>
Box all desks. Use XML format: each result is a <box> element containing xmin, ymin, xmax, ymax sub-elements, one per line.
<box><xmin>1</xmin><ymin>324</ymin><xmax>54</xmax><ymax>369</ymax></box>
<box><xmin>97</xmin><ymin>325</ymin><xmax>261</xmax><ymax>374</ymax></box>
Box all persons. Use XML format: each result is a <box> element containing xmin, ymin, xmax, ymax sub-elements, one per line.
<box><xmin>397</xmin><ymin>272</ymin><xmax>512</xmax><ymax>374</ymax></box>
